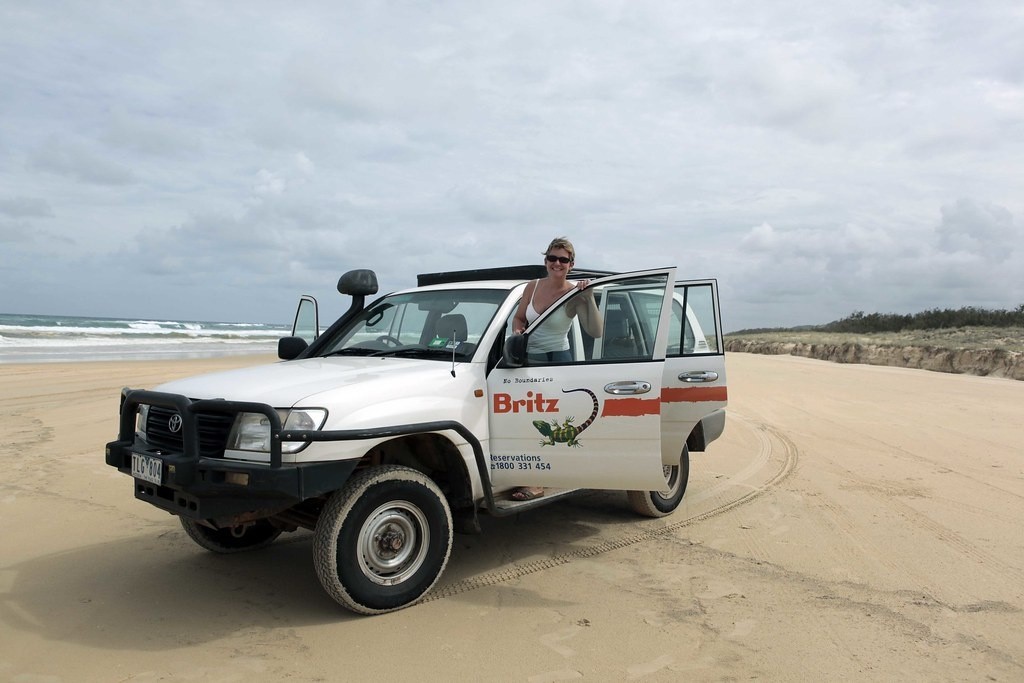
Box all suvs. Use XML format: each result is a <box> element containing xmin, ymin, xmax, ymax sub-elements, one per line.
<box><xmin>104</xmin><ymin>262</ymin><xmax>727</xmax><ymax>615</ymax></box>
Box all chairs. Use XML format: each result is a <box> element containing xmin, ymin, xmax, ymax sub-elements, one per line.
<box><xmin>602</xmin><ymin>311</ymin><xmax>630</xmax><ymax>358</ymax></box>
<box><xmin>435</xmin><ymin>314</ymin><xmax>474</xmax><ymax>352</ymax></box>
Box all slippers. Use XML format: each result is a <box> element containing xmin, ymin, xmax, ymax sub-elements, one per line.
<box><xmin>513</xmin><ymin>488</ymin><xmax>544</xmax><ymax>500</ymax></box>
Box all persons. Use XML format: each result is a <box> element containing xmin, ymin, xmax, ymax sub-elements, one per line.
<box><xmin>511</xmin><ymin>237</ymin><xmax>605</xmax><ymax>500</ymax></box>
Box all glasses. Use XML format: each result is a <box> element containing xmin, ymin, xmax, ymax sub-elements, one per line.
<box><xmin>546</xmin><ymin>254</ymin><xmax>572</xmax><ymax>263</ymax></box>
